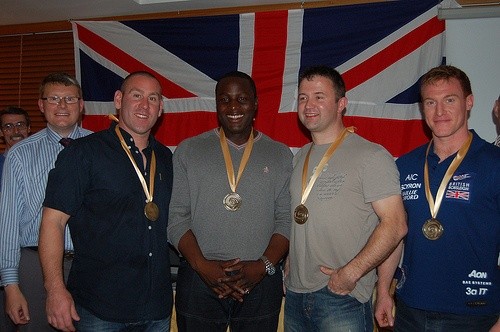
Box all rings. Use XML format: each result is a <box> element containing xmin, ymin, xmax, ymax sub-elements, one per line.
<box><xmin>245</xmin><ymin>289</ymin><xmax>249</xmax><ymax>294</ymax></box>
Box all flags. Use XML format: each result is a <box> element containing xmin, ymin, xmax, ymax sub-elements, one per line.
<box><xmin>69</xmin><ymin>0</ymin><xmax>445</xmax><ymax>162</ymax></box>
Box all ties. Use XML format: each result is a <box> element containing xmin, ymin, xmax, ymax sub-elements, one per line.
<box><xmin>59</xmin><ymin>138</ymin><xmax>74</xmax><ymax>148</ymax></box>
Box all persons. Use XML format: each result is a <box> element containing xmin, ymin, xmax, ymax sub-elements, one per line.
<box><xmin>167</xmin><ymin>72</ymin><xmax>294</xmax><ymax>332</ymax></box>
<box><xmin>0</xmin><ymin>107</ymin><xmax>31</xmax><ymax>189</ymax></box>
<box><xmin>40</xmin><ymin>71</ymin><xmax>173</xmax><ymax>332</ymax></box>
<box><xmin>0</xmin><ymin>73</ymin><xmax>97</xmax><ymax>332</ymax></box>
<box><xmin>283</xmin><ymin>67</ymin><xmax>407</xmax><ymax>332</ymax></box>
<box><xmin>376</xmin><ymin>64</ymin><xmax>500</xmax><ymax>332</ymax></box>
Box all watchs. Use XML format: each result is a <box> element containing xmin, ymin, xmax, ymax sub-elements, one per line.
<box><xmin>260</xmin><ymin>256</ymin><xmax>276</xmax><ymax>275</ymax></box>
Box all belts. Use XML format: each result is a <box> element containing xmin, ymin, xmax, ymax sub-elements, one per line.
<box><xmin>24</xmin><ymin>246</ymin><xmax>75</xmax><ymax>258</ymax></box>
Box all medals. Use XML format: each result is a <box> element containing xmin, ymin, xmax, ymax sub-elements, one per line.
<box><xmin>144</xmin><ymin>203</ymin><xmax>159</xmax><ymax>221</ymax></box>
<box><xmin>222</xmin><ymin>193</ymin><xmax>242</xmax><ymax>212</ymax></box>
<box><xmin>293</xmin><ymin>204</ymin><xmax>309</xmax><ymax>224</ymax></box>
<box><xmin>422</xmin><ymin>219</ymin><xmax>444</xmax><ymax>240</ymax></box>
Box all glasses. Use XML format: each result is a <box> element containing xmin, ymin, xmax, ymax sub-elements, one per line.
<box><xmin>2</xmin><ymin>121</ymin><xmax>27</xmax><ymax>130</ymax></box>
<box><xmin>41</xmin><ymin>94</ymin><xmax>81</xmax><ymax>104</ymax></box>
